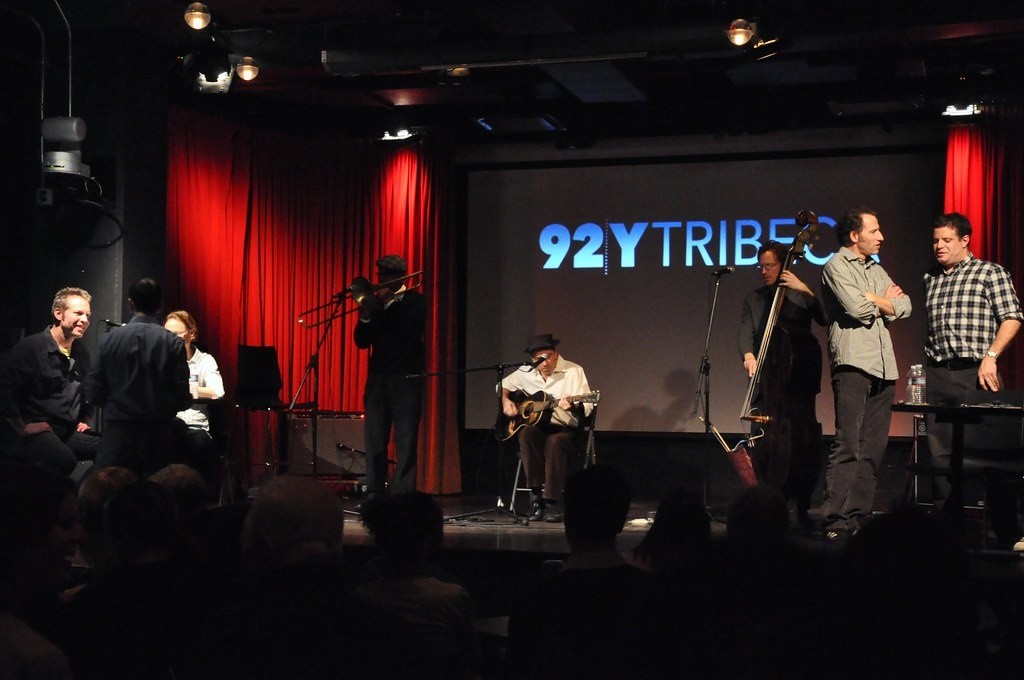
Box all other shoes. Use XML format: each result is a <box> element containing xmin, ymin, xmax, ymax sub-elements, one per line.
<box><xmin>543</xmin><ymin>499</ymin><xmax>563</xmax><ymax>522</ymax></box>
<box><xmin>1013</xmin><ymin>536</ymin><xmax>1024</xmax><ymax>551</ymax></box>
<box><xmin>528</xmin><ymin>498</ymin><xmax>545</xmax><ymax>521</ymax></box>
<box><xmin>353</xmin><ymin>499</ymin><xmax>371</xmax><ymax>512</ymax></box>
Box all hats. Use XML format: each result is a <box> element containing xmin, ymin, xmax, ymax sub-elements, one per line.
<box><xmin>376</xmin><ymin>255</ymin><xmax>407</xmax><ymax>274</ymax></box>
<box><xmin>524</xmin><ymin>334</ymin><xmax>561</xmax><ymax>354</ymax></box>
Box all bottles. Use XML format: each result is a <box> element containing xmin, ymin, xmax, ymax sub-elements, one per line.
<box><xmin>911</xmin><ymin>363</ymin><xmax>927</xmax><ymax>405</ymax></box>
<box><xmin>904</xmin><ymin>365</ymin><xmax>917</xmax><ymax>406</ymax></box>
<box><xmin>189</xmin><ymin>361</ymin><xmax>199</xmax><ymax>399</ymax></box>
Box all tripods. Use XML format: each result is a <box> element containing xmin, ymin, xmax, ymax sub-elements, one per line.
<box><xmin>406</xmin><ymin>361</ymin><xmax>534</xmax><ymax>526</ymax></box>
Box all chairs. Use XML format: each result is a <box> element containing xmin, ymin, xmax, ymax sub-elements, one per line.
<box><xmin>509</xmin><ymin>390</ymin><xmax>602</xmax><ymax>523</ymax></box>
<box><xmin>209</xmin><ymin>400</ymin><xmax>237</xmax><ymax>508</ymax></box>
<box><xmin>903</xmin><ymin>417</ymin><xmax>990</xmax><ymax>550</ymax></box>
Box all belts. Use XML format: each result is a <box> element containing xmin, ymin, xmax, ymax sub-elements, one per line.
<box><xmin>929</xmin><ymin>355</ymin><xmax>981</xmax><ymax>372</ymax></box>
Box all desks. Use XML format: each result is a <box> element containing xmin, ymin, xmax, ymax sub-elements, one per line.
<box><xmin>892</xmin><ymin>403</ymin><xmax>1024</xmax><ymax>548</ymax></box>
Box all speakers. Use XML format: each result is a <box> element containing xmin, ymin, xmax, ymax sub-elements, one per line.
<box><xmin>277</xmin><ymin>410</ymin><xmax>367</xmax><ymax>478</ymax></box>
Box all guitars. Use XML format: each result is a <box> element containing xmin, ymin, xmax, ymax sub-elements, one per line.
<box><xmin>495</xmin><ymin>385</ymin><xmax>601</xmax><ymax>442</ymax></box>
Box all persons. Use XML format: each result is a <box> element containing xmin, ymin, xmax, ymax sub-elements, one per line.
<box><xmin>0</xmin><ymin>287</ymin><xmax>102</xmax><ymax>488</ymax></box>
<box><xmin>821</xmin><ymin>207</ymin><xmax>913</xmax><ymax>543</ymax></box>
<box><xmin>921</xmin><ymin>213</ymin><xmax>1024</xmax><ymax>551</ymax></box>
<box><xmin>163</xmin><ymin>309</ymin><xmax>225</xmax><ymax>482</ymax></box>
<box><xmin>354</xmin><ymin>254</ymin><xmax>427</xmax><ymax>511</ymax></box>
<box><xmin>494</xmin><ymin>333</ymin><xmax>594</xmax><ymax>522</ymax></box>
<box><xmin>87</xmin><ymin>276</ymin><xmax>193</xmax><ymax>475</ymax></box>
<box><xmin>737</xmin><ymin>240</ymin><xmax>828</xmax><ymax>536</ymax></box>
<box><xmin>0</xmin><ymin>459</ymin><xmax>1024</xmax><ymax>680</ymax></box>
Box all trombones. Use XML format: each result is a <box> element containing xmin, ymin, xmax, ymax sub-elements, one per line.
<box><xmin>298</xmin><ymin>270</ymin><xmax>429</xmax><ymax>329</ymax></box>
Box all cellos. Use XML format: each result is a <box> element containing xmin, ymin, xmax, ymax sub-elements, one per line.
<box><xmin>749</xmin><ymin>208</ymin><xmax>823</xmax><ymax>497</ymax></box>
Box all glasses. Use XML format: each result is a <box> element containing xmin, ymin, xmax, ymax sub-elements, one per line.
<box><xmin>176</xmin><ymin>327</ymin><xmax>190</xmax><ymax>338</ymax></box>
<box><xmin>376</xmin><ymin>270</ymin><xmax>397</xmax><ymax>278</ymax></box>
<box><xmin>756</xmin><ymin>261</ymin><xmax>782</xmax><ymax>271</ymax></box>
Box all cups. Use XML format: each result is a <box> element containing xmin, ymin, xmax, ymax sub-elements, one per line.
<box><xmin>647</xmin><ymin>511</ymin><xmax>656</xmax><ymax>524</ymax></box>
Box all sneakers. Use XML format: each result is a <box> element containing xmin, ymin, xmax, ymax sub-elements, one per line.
<box><xmin>823</xmin><ymin>527</ymin><xmax>848</xmax><ymax>544</ymax></box>
<box><xmin>846</xmin><ymin>527</ymin><xmax>862</xmax><ymax>539</ymax></box>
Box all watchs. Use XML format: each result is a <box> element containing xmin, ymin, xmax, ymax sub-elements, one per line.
<box><xmin>986</xmin><ymin>350</ymin><xmax>998</xmax><ymax>359</ymax></box>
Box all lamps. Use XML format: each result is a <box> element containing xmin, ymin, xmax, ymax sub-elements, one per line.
<box><xmin>940</xmin><ymin>74</ymin><xmax>986</xmax><ymax>117</ymax></box>
<box><xmin>722</xmin><ymin>17</ymin><xmax>779</xmax><ymax>50</ymax></box>
<box><xmin>373</xmin><ymin>106</ymin><xmax>420</xmax><ymax>148</ymax></box>
<box><xmin>179</xmin><ymin>2</ymin><xmax>260</xmax><ymax>94</ymax></box>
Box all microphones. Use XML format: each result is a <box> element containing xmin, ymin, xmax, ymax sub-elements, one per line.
<box><xmin>331</xmin><ymin>286</ymin><xmax>356</xmax><ymax>299</ymax></box>
<box><xmin>102</xmin><ymin>318</ymin><xmax>126</xmax><ymax>327</ymax></box>
<box><xmin>528</xmin><ymin>353</ymin><xmax>548</xmax><ymax>371</ymax></box>
<box><xmin>338</xmin><ymin>443</ymin><xmax>355</xmax><ymax>451</ymax></box>
<box><xmin>712</xmin><ymin>266</ymin><xmax>735</xmax><ymax>275</ymax></box>
